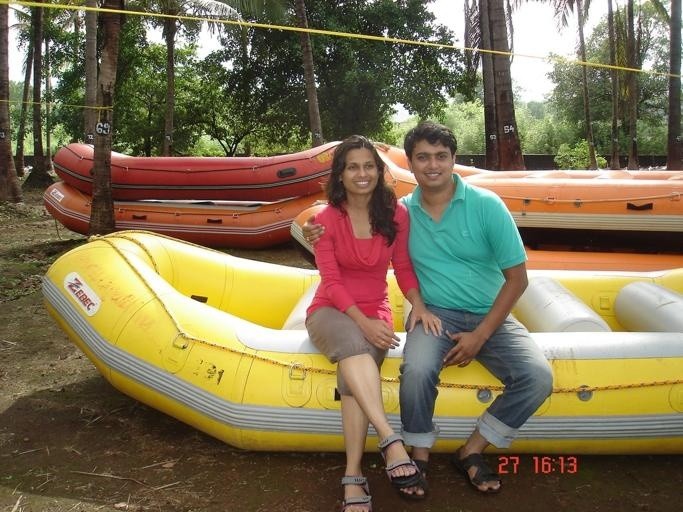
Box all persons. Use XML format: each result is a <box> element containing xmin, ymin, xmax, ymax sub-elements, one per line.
<box><xmin>301</xmin><ymin>121</ymin><xmax>553</xmax><ymax>500</ymax></box>
<box><xmin>306</xmin><ymin>134</ymin><xmax>444</xmax><ymax>512</ymax></box>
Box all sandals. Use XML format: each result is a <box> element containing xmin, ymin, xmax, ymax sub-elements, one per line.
<box><xmin>454</xmin><ymin>447</ymin><xmax>502</xmax><ymax>494</ymax></box>
<box><xmin>377</xmin><ymin>433</ymin><xmax>420</xmax><ymax>487</ymax></box>
<box><xmin>341</xmin><ymin>476</ymin><xmax>372</xmax><ymax>512</ymax></box>
<box><xmin>399</xmin><ymin>457</ymin><xmax>429</xmax><ymax>498</ymax></box>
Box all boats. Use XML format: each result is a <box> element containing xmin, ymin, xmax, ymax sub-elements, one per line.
<box><xmin>43</xmin><ymin>142</ymin><xmax>336</xmax><ymax>250</ymax></box>
<box><xmin>42</xmin><ymin>228</ymin><xmax>683</xmax><ymax>455</ymax></box>
<box><xmin>375</xmin><ymin>144</ymin><xmax>682</xmax><ymax>253</ymax></box>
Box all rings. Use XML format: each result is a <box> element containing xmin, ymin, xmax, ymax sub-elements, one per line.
<box><xmin>306</xmin><ymin>236</ymin><xmax>310</xmax><ymax>242</ymax></box>
